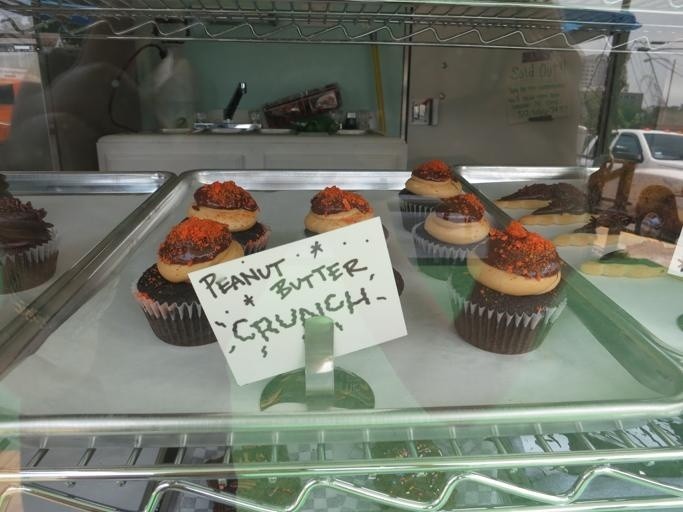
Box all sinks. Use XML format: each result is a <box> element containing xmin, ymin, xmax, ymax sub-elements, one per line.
<box><xmin>154</xmin><ymin>126</ymin><xmax>194</xmax><ymax>135</ymax></box>
<box><xmin>195</xmin><ymin>123</ymin><xmax>262</xmax><ymax>134</ymax></box>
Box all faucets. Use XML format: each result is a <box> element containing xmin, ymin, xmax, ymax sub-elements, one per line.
<box><xmin>223</xmin><ymin>80</ymin><xmax>248</xmax><ymax>126</ymax></box>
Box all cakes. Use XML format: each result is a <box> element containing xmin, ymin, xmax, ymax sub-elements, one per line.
<box><xmin>305</xmin><ymin>186</ymin><xmax>375</xmax><ymax>232</ymax></box>
<box><xmin>133</xmin><ymin>216</ymin><xmax>244</xmax><ymax>347</ymax></box>
<box><xmin>398</xmin><ymin>160</ymin><xmax>462</xmax><ymax>229</ymax></box>
<box><xmin>495</xmin><ymin>183</ymin><xmax>578</xmax><ymax>208</ymax></box>
<box><xmin>0</xmin><ymin>195</ymin><xmax>59</xmax><ymax>293</ymax></box>
<box><xmin>578</xmin><ymin>238</ymin><xmax>669</xmax><ymax>279</ymax></box>
<box><xmin>412</xmin><ymin>194</ymin><xmax>485</xmax><ymax>281</ymax></box>
<box><xmin>182</xmin><ymin>181</ymin><xmax>271</xmax><ymax>257</ymax></box>
<box><xmin>556</xmin><ymin>214</ymin><xmax>623</xmax><ymax>245</ymax></box>
<box><xmin>520</xmin><ymin>193</ymin><xmax>587</xmax><ymax>225</ymax></box>
<box><xmin>447</xmin><ymin>219</ymin><xmax>569</xmax><ymax>354</ymax></box>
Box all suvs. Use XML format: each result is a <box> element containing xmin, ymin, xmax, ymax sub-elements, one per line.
<box><xmin>580</xmin><ymin>128</ymin><xmax>683</xmax><ymax>243</ymax></box>
